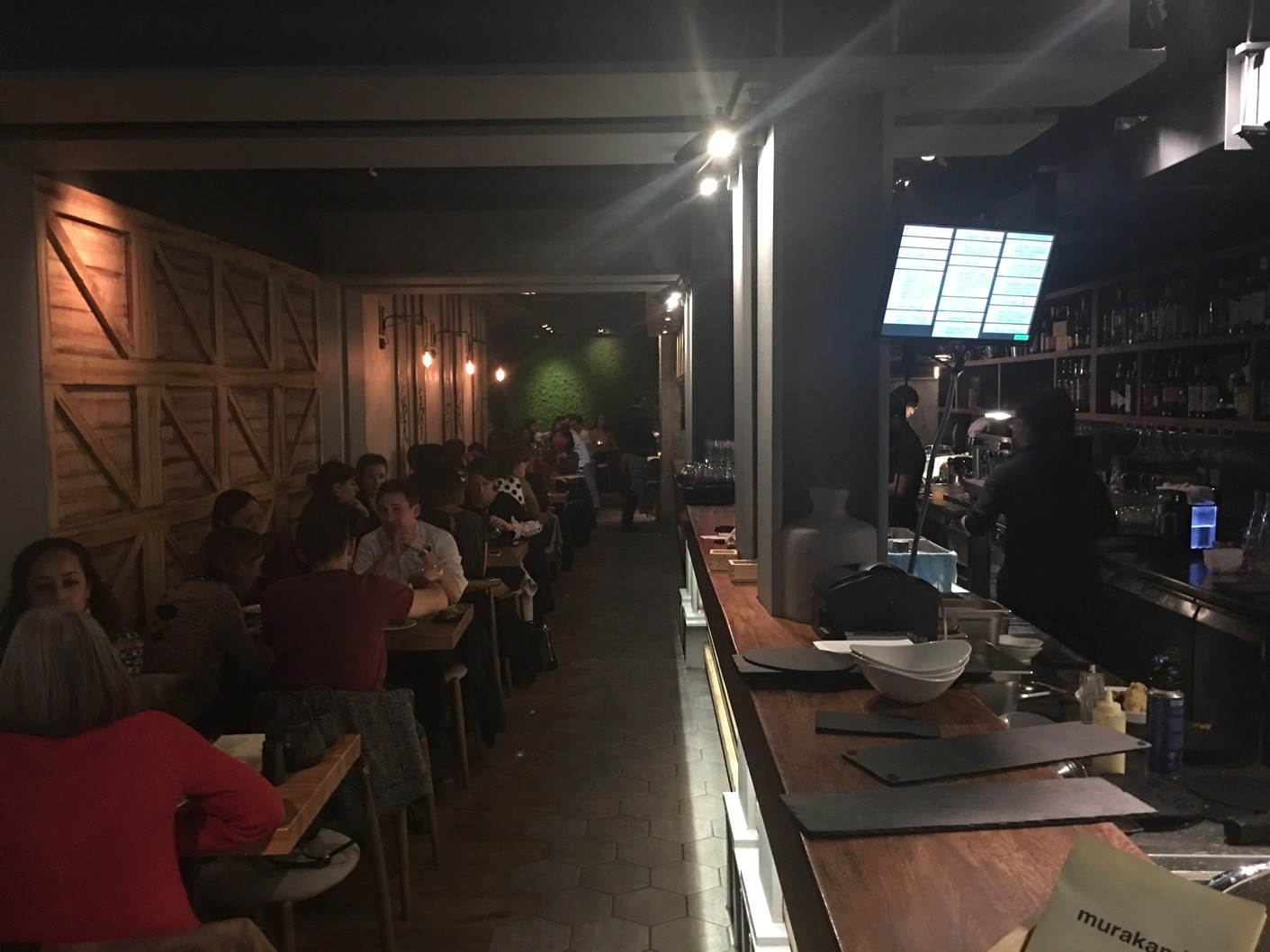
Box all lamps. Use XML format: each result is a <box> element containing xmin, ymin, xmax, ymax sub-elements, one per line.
<box><xmin>377</xmin><ymin>305</ymin><xmax>433</xmax><ymax>370</ymax></box>
<box><xmin>653</xmin><ymin>277</ymin><xmax>690</xmax><ymax>335</ymax></box>
<box><xmin>670</xmin><ymin>87</ymin><xmax>770</xmax><ymax>196</ymax></box>
<box><xmin>466</xmin><ymin>339</ymin><xmax>504</xmax><ymax>383</ymax></box>
<box><xmin>430</xmin><ymin>323</ymin><xmax>474</xmax><ymax>375</ymax></box>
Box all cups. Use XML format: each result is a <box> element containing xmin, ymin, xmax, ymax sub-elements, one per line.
<box><xmin>483</xmin><ymin>515</ymin><xmax>501</xmax><ymax>539</ymax></box>
<box><xmin>1075</xmin><ymin>670</ymin><xmax>1106</xmax><ymax>724</ymax></box>
<box><xmin>682</xmin><ymin>436</ymin><xmax>735</xmax><ymax>489</ymax></box>
<box><xmin>1188</xmin><ymin>503</ymin><xmax>1218</xmax><ymax>550</ymax></box>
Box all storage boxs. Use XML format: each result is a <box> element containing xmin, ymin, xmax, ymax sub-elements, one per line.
<box><xmin>673</xmin><ymin>472</ymin><xmax>735</xmax><ymax>507</ymax></box>
<box><xmin>887</xmin><ymin>526</ymin><xmax>958</xmax><ymax>594</ymax></box>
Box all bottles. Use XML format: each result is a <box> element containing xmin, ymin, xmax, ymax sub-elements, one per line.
<box><xmin>1245</xmin><ymin>488</ymin><xmax>1270</xmax><ymax>582</ymax></box>
<box><xmin>1087</xmin><ymin>686</ymin><xmax>1128</xmax><ymax>778</ymax></box>
<box><xmin>986</xmin><ymin>274</ymin><xmax>1270</xmax><ymax>532</ymax></box>
<box><xmin>1148</xmin><ymin>657</ymin><xmax>1185</xmax><ymax>776</ymax></box>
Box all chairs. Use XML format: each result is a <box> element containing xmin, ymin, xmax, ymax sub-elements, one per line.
<box><xmin>238</xmin><ymin>602</ymin><xmax>477</xmax><ymax>788</ymax></box>
<box><xmin>245</xmin><ymin>689</ymin><xmax>441</xmax><ymax>923</ymax></box>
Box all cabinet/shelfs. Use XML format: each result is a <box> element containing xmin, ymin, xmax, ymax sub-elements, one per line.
<box><xmin>912</xmin><ymin>479</ymin><xmax>1270</xmax><ymax>817</ymax></box>
<box><xmin>939</xmin><ymin>237</ymin><xmax>1270</xmax><ymax>524</ymax></box>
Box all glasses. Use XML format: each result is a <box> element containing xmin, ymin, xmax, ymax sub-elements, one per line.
<box><xmin>1008</xmin><ymin>418</ymin><xmax>1026</xmax><ymax>429</ymax></box>
<box><xmin>906</xmin><ymin>402</ymin><xmax>918</xmax><ymax>410</ymax></box>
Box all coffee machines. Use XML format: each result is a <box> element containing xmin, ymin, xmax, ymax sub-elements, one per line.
<box><xmin>961</xmin><ymin>411</ymin><xmax>1022</xmax><ymax>504</ymax></box>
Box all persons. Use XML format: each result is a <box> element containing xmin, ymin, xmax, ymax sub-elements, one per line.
<box><xmin>887</xmin><ymin>393</ymin><xmax>926</xmax><ymax>532</ymax></box>
<box><xmin>0</xmin><ymin>537</ymin><xmax>120</xmax><ymax>667</ymax></box>
<box><xmin>891</xmin><ymin>385</ymin><xmax>919</xmax><ymax>418</ymax></box>
<box><xmin>140</xmin><ymin>388</ymin><xmax>659</xmax><ymax>836</ymax></box>
<box><xmin>963</xmin><ymin>387</ymin><xmax>1118</xmax><ymax>668</ymax></box>
<box><xmin>0</xmin><ymin>601</ymin><xmax>286</xmax><ymax>945</ymax></box>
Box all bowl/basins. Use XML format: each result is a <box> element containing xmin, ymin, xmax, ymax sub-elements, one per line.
<box><xmin>849</xmin><ymin>639</ymin><xmax>971</xmax><ymax>704</ymax></box>
<box><xmin>1000</xmin><ymin>634</ymin><xmax>1045</xmax><ymax>664</ymax></box>
<box><xmin>1203</xmin><ymin>548</ymin><xmax>1244</xmax><ymax>574</ymax></box>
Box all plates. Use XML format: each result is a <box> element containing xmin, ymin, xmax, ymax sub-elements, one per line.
<box><xmin>1071</xmin><ymin>685</ymin><xmax>1146</xmax><ymax>726</ymax></box>
<box><xmin>243</xmin><ymin>604</ymin><xmax>262</xmax><ymax>614</ymax></box>
<box><xmin>385</xmin><ymin>619</ymin><xmax>417</xmax><ymax>631</ymax></box>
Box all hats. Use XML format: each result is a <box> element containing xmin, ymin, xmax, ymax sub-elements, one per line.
<box><xmin>891</xmin><ymin>384</ymin><xmax>919</xmax><ymax>403</ymax></box>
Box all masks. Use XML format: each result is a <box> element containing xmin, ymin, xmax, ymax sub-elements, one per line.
<box><xmin>1011</xmin><ymin>435</ymin><xmax>1029</xmax><ymax>451</ymax></box>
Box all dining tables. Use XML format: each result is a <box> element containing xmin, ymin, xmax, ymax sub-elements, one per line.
<box><xmin>483</xmin><ymin>454</ymin><xmax>660</xmax><ymax>699</ymax></box>
<box><xmin>171</xmin><ymin>720</ymin><xmax>395</xmax><ymax>952</ymax></box>
